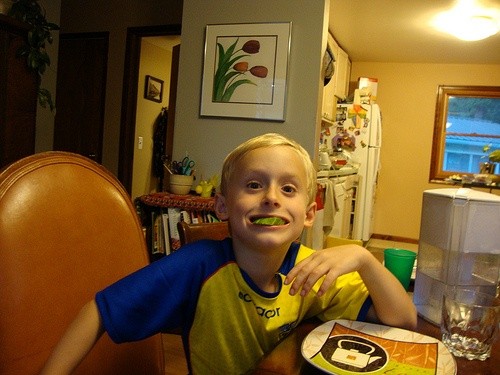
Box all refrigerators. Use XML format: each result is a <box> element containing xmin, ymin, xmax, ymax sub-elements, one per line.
<box><xmin>334</xmin><ymin>100</ymin><xmax>381</xmax><ymax>242</ymax></box>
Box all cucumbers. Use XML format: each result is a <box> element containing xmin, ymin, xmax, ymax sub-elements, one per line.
<box><xmin>252</xmin><ymin>217</ymin><xmax>286</xmax><ymax>225</ymax></box>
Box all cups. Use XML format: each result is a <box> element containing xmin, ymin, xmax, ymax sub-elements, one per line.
<box><xmin>383</xmin><ymin>248</ymin><xmax>417</xmax><ymax>293</ymax></box>
<box><xmin>440</xmin><ymin>286</ymin><xmax>500</xmax><ymax>361</ymax></box>
<box><xmin>169</xmin><ymin>174</ymin><xmax>194</xmax><ymax>195</ymax></box>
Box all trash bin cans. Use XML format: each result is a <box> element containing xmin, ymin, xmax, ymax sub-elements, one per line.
<box><xmin>384</xmin><ymin>249</ymin><xmax>417</xmax><ymax>291</ymax></box>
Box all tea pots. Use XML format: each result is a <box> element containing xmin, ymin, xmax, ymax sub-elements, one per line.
<box><xmin>319</xmin><ymin>151</ymin><xmax>331</xmax><ymax>170</ymax></box>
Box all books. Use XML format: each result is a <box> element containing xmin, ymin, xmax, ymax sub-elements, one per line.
<box><xmin>151</xmin><ymin>207</ymin><xmax>224</xmax><ymax>255</ymax></box>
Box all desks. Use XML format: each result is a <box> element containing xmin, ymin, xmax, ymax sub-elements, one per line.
<box><xmin>134</xmin><ymin>192</ymin><xmax>225</xmax><ymax>262</ymax></box>
<box><xmin>249</xmin><ymin>289</ymin><xmax>500</xmax><ymax>375</ymax></box>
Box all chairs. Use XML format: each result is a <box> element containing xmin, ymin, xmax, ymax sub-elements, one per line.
<box><xmin>176</xmin><ymin>216</ymin><xmax>234</xmax><ymax>250</ymax></box>
<box><xmin>0</xmin><ymin>149</ymin><xmax>166</xmax><ymax>375</ymax></box>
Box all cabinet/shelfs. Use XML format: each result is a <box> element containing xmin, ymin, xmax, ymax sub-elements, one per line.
<box><xmin>315</xmin><ymin>174</ymin><xmax>355</xmax><ymax>249</ymax></box>
<box><xmin>321</xmin><ymin>30</ymin><xmax>352</xmax><ymax>124</ymax></box>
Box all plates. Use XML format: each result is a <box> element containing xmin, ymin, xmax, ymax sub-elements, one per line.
<box><xmin>300</xmin><ymin>318</ymin><xmax>457</xmax><ymax>375</ymax></box>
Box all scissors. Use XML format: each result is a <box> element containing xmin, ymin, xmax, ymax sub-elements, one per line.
<box><xmin>183</xmin><ymin>157</ymin><xmax>194</xmax><ymax>168</ymax></box>
<box><xmin>173</xmin><ymin>160</ymin><xmax>183</xmax><ymax>174</ymax></box>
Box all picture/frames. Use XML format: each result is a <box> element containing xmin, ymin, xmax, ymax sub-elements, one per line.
<box><xmin>196</xmin><ymin>20</ymin><xmax>294</xmax><ymax>125</ymax></box>
<box><xmin>143</xmin><ymin>75</ymin><xmax>165</xmax><ymax>104</ymax></box>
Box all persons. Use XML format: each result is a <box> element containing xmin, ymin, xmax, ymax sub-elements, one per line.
<box><xmin>39</xmin><ymin>134</ymin><xmax>418</xmax><ymax>375</ymax></box>
